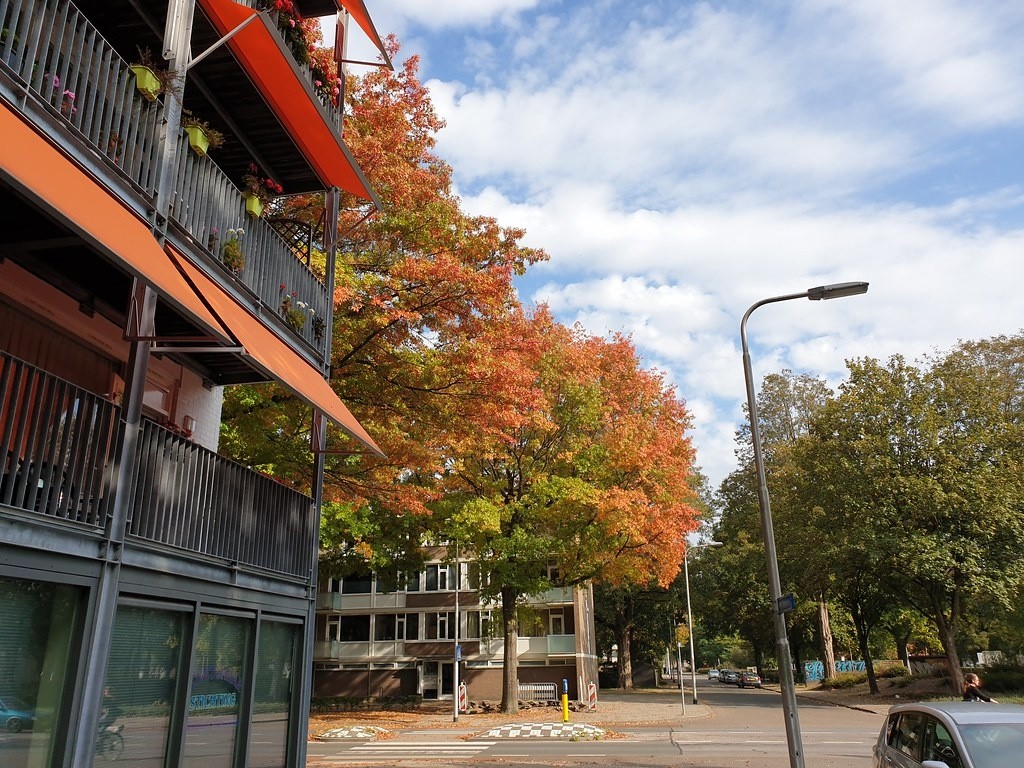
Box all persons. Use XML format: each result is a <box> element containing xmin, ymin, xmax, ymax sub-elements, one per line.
<box><xmin>963</xmin><ymin>672</ymin><xmax>998</xmax><ymax>704</ymax></box>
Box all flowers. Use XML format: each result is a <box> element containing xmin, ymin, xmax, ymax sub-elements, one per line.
<box><xmin>202</xmin><ymin>224</ymin><xmax>316</xmax><ymax>331</ymax></box>
<box><xmin>46</xmin><ymin>73</ymin><xmax>78</xmax><ymax>114</ymax></box>
<box><xmin>241</xmin><ymin>163</ymin><xmax>283</xmax><ymax>209</ymax></box>
<box><xmin>259</xmin><ymin>0</ymin><xmax>342</xmax><ymax>106</ymax></box>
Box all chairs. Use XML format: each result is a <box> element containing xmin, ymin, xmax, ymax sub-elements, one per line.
<box><xmin>0</xmin><ymin>446</ymin><xmax>76</xmax><ymax>516</ymax></box>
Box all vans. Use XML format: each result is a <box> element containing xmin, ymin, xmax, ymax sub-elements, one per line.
<box><xmin>718</xmin><ymin>668</ymin><xmax>731</xmax><ymax>682</ymax></box>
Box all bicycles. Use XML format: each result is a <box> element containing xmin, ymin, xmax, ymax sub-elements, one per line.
<box><xmin>94</xmin><ymin>711</ymin><xmax>125</xmax><ymax>761</ymax></box>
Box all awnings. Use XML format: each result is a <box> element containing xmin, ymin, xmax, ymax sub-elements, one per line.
<box><xmin>195</xmin><ymin>0</ymin><xmax>394</xmax><ymax>211</ymax></box>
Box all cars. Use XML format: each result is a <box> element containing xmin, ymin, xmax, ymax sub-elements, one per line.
<box><xmin>872</xmin><ymin>701</ymin><xmax>1024</xmax><ymax>768</ymax></box>
<box><xmin>0</xmin><ymin>695</ymin><xmax>54</xmax><ymax>733</ymax></box>
<box><xmin>724</xmin><ymin>672</ymin><xmax>739</xmax><ymax>684</ymax></box>
<box><xmin>736</xmin><ymin>670</ymin><xmax>762</xmax><ymax>690</ymax></box>
<box><xmin>708</xmin><ymin>669</ymin><xmax>720</xmax><ymax>680</ymax></box>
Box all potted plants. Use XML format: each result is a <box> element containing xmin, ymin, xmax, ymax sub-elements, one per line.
<box><xmin>181</xmin><ymin>116</ymin><xmax>225</xmax><ymax>156</ymax></box>
<box><xmin>130</xmin><ymin>45</ymin><xmax>192</xmax><ymax>115</ymax></box>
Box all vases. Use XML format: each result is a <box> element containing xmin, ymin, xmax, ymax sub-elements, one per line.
<box><xmin>246</xmin><ymin>192</ymin><xmax>265</xmax><ymax>219</ymax></box>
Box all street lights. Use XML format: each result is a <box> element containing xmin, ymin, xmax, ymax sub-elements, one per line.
<box><xmin>684</xmin><ymin>542</ymin><xmax>724</xmax><ymax>704</ymax></box>
<box><xmin>740</xmin><ymin>281</ymin><xmax>869</xmax><ymax>768</ymax></box>
